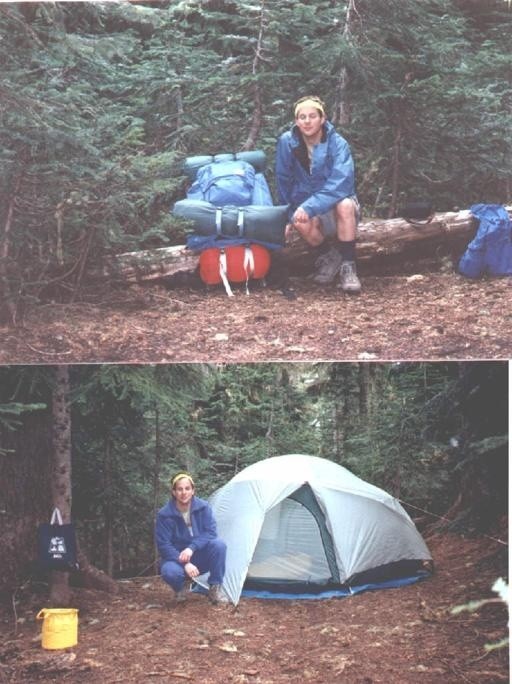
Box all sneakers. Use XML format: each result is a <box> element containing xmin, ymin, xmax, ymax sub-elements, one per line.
<box><xmin>312</xmin><ymin>245</ymin><xmax>343</xmax><ymax>284</ymax></box>
<box><xmin>172</xmin><ymin>588</ymin><xmax>187</xmax><ymax>604</ymax></box>
<box><xmin>339</xmin><ymin>261</ymin><xmax>362</xmax><ymax>292</ymax></box>
<box><xmin>208</xmin><ymin>589</ymin><xmax>228</xmax><ymax>607</ymax></box>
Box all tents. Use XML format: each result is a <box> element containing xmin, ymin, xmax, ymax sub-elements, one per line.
<box><xmin>206</xmin><ymin>453</ymin><xmax>434</xmax><ymax>611</ymax></box>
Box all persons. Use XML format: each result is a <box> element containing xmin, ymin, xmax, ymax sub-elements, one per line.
<box><xmin>156</xmin><ymin>474</ymin><xmax>229</xmax><ymax>605</ymax></box>
<box><xmin>276</xmin><ymin>95</ymin><xmax>364</xmax><ymax>289</ymax></box>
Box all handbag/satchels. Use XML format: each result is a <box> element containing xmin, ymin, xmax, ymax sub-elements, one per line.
<box><xmin>37</xmin><ymin>506</ymin><xmax>78</xmax><ymax>574</ymax></box>
<box><xmin>398</xmin><ymin>199</ymin><xmax>436</xmax><ymax>228</ymax></box>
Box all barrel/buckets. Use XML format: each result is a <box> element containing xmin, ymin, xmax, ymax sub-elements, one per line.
<box><xmin>37</xmin><ymin>607</ymin><xmax>80</xmax><ymax>651</ymax></box>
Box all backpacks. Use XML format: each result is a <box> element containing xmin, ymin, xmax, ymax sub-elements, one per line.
<box><xmin>197</xmin><ymin>242</ymin><xmax>274</xmax><ymax>298</ymax></box>
<box><xmin>171</xmin><ymin>199</ymin><xmax>293</xmax><ymax>246</ymax></box>
<box><xmin>197</xmin><ymin>161</ymin><xmax>257</xmax><ymax>206</ymax></box>
